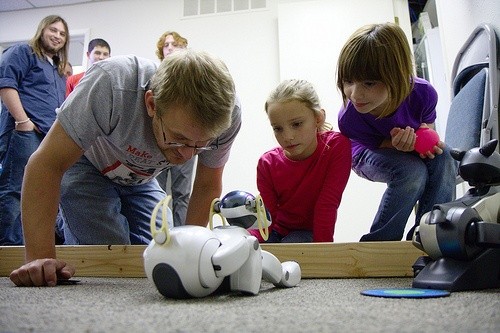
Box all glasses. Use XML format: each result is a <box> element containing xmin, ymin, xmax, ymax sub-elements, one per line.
<box><xmin>161</xmin><ymin>114</ymin><xmax>220</xmax><ymax>151</ymax></box>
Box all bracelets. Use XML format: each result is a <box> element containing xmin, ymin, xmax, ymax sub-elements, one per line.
<box><xmin>15</xmin><ymin>118</ymin><xmax>30</xmax><ymax>125</ymax></box>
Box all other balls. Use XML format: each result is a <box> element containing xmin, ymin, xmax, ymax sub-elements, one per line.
<box><xmin>413</xmin><ymin>127</ymin><xmax>440</xmax><ymax>155</ymax></box>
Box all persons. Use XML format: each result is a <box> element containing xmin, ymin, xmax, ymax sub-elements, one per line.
<box><xmin>247</xmin><ymin>80</ymin><xmax>352</xmax><ymax>243</ymax></box>
<box><xmin>155</xmin><ymin>31</ymin><xmax>196</xmax><ymax>227</ymax></box>
<box><xmin>336</xmin><ymin>21</ymin><xmax>457</xmax><ymax>242</ymax></box>
<box><xmin>0</xmin><ymin>14</ymin><xmax>70</xmax><ymax>247</ymax></box>
<box><xmin>58</xmin><ymin>39</ymin><xmax>111</xmax><ymax>98</ymax></box>
<box><xmin>10</xmin><ymin>49</ymin><xmax>242</xmax><ymax>287</ymax></box>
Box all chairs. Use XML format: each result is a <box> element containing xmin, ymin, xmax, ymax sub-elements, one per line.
<box><xmin>444</xmin><ymin>23</ymin><xmax>500</xmax><ymax>200</ymax></box>
